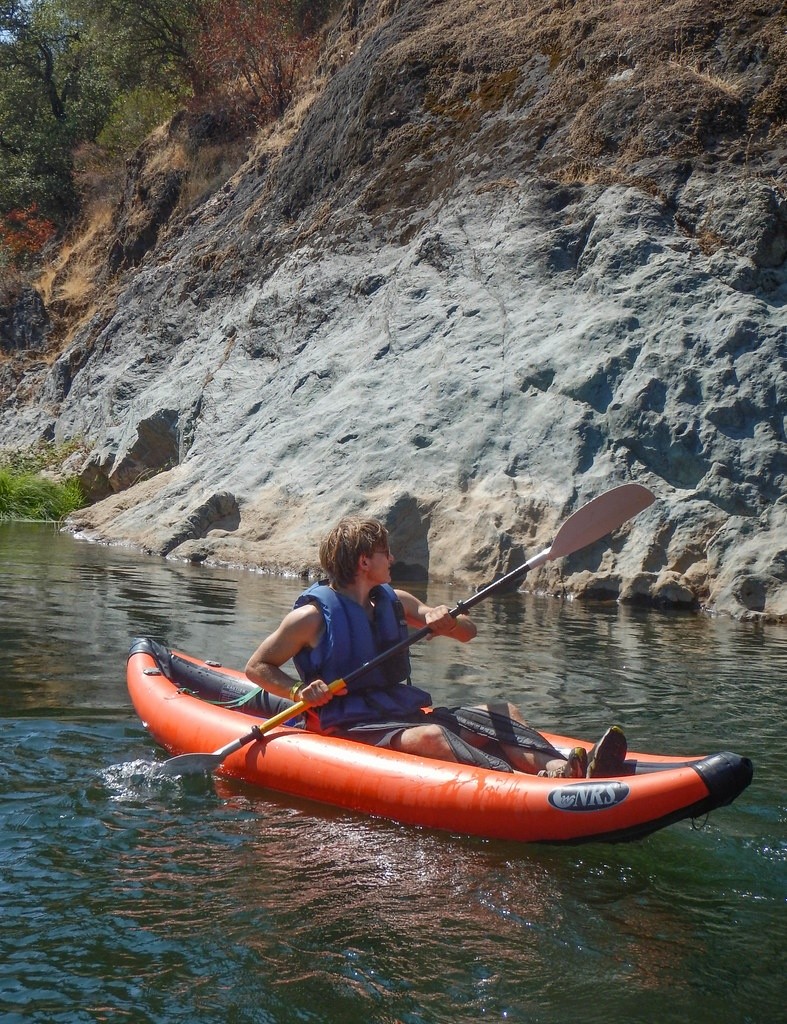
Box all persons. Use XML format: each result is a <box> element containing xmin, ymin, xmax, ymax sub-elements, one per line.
<box><xmin>245</xmin><ymin>516</ymin><xmax>628</xmax><ymax>778</ymax></box>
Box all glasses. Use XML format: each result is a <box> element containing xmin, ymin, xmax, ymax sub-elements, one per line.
<box><xmin>376</xmin><ymin>546</ymin><xmax>391</xmax><ymax>555</ymax></box>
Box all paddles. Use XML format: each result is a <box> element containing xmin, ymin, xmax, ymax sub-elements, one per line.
<box><xmin>161</xmin><ymin>482</ymin><xmax>657</xmax><ymax>774</ymax></box>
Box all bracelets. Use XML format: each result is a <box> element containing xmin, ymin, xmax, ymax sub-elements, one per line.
<box><xmin>290</xmin><ymin>681</ymin><xmax>304</xmax><ymax>702</ymax></box>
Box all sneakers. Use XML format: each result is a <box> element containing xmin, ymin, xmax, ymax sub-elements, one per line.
<box><xmin>537</xmin><ymin>747</ymin><xmax>589</xmax><ymax>778</ymax></box>
<box><xmin>586</xmin><ymin>726</ymin><xmax>627</xmax><ymax>778</ymax></box>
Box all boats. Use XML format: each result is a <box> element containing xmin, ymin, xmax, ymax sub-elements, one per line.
<box><xmin>123</xmin><ymin>635</ymin><xmax>753</xmax><ymax>844</ymax></box>
